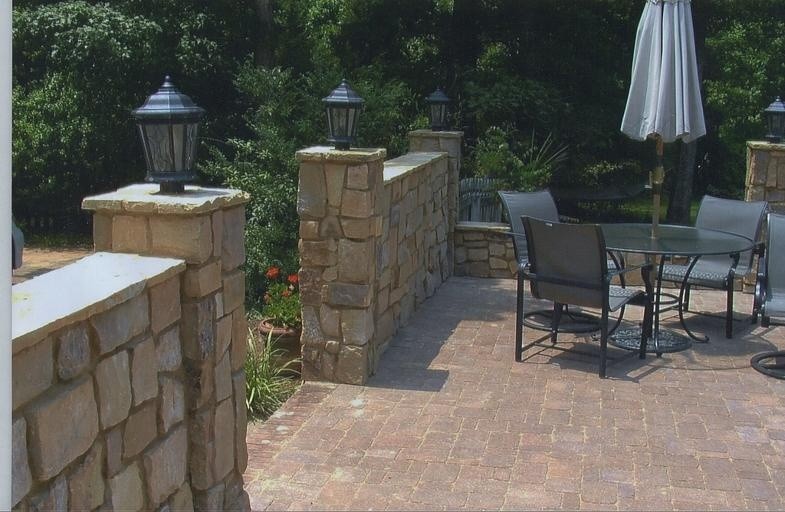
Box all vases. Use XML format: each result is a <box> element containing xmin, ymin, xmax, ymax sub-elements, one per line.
<box><xmin>260</xmin><ymin>320</ymin><xmax>299</xmax><ymax>372</ymax></box>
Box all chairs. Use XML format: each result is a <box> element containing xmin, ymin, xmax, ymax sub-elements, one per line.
<box><xmin>511</xmin><ymin>215</ymin><xmax>643</xmax><ymax>378</ymax></box>
<box><xmin>501</xmin><ymin>182</ymin><xmax>620</xmax><ymax>333</ymax></box>
<box><xmin>651</xmin><ymin>193</ymin><xmax>767</xmax><ymax>340</ymax></box>
<box><xmin>752</xmin><ymin>211</ymin><xmax>785</xmax><ymax>381</ymax></box>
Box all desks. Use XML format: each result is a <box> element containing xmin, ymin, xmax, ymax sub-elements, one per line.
<box><xmin>582</xmin><ymin>225</ymin><xmax>753</xmax><ymax>351</ymax></box>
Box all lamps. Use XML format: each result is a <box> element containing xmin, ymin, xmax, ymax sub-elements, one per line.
<box><xmin>762</xmin><ymin>96</ymin><xmax>785</xmax><ymax>143</ymax></box>
<box><xmin>324</xmin><ymin>73</ymin><xmax>363</xmax><ymax>149</ymax></box>
<box><xmin>426</xmin><ymin>83</ymin><xmax>453</xmax><ymax>131</ymax></box>
<box><xmin>128</xmin><ymin>74</ymin><xmax>206</xmax><ymax>194</ymax></box>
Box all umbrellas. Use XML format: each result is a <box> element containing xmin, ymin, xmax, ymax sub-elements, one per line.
<box><xmin>620</xmin><ymin>0</ymin><xmax>707</xmax><ymax>339</ymax></box>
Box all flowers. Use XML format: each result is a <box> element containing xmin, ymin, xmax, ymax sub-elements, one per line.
<box><xmin>262</xmin><ymin>265</ymin><xmax>299</xmax><ymax>327</ymax></box>
